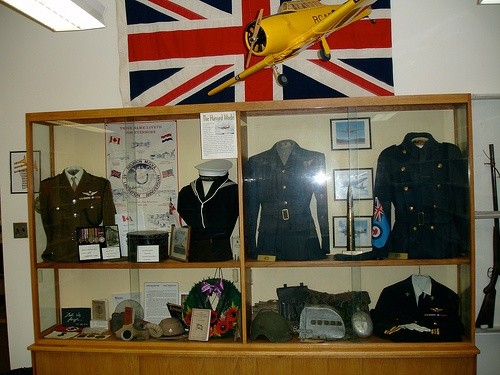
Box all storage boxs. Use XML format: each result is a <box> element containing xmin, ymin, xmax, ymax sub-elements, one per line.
<box><xmin>127</xmin><ymin>230</ymin><xmax>169</xmax><ymax>262</ymax></box>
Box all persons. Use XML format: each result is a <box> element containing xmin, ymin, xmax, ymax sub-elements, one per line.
<box><xmin>245</xmin><ymin>139</ymin><xmax>330</xmax><ymax>259</ymax></box>
<box><xmin>375</xmin><ymin>131</ymin><xmax>467</xmax><ymax>257</ymax></box>
<box><xmin>178</xmin><ymin>159</ymin><xmax>240</xmax><ymax>263</ymax></box>
<box><xmin>40</xmin><ymin>167</ymin><xmax>115</xmax><ymax>262</ymax></box>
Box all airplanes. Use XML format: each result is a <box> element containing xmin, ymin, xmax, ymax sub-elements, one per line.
<box><xmin>207</xmin><ymin>0</ymin><xmax>377</xmax><ymax>96</ymax></box>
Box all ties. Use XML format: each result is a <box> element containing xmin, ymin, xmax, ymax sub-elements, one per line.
<box><xmin>71</xmin><ymin>177</ymin><xmax>78</xmax><ymax>191</ymax></box>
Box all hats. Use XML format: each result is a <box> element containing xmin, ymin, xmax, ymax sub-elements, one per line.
<box><xmin>195</xmin><ymin>159</ymin><xmax>233</xmax><ymax>181</ymax></box>
<box><xmin>251</xmin><ymin>309</ymin><xmax>292</xmax><ymax>342</ymax></box>
<box><xmin>157</xmin><ymin>318</ymin><xmax>186</xmax><ymax>339</ymax></box>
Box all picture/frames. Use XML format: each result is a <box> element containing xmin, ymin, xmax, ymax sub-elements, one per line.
<box><xmin>9</xmin><ymin>150</ymin><xmax>41</xmax><ymax>194</ymax></box>
<box><xmin>329</xmin><ymin>117</ymin><xmax>371</xmax><ymax>150</ymax></box>
<box><xmin>169</xmin><ymin>223</ymin><xmax>190</xmax><ymax>264</ymax></box>
<box><xmin>332</xmin><ymin>216</ymin><xmax>372</xmax><ymax>248</ymax></box>
<box><xmin>333</xmin><ymin>168</ymin><xmax>374</xmax><ymax>201</ymax></box>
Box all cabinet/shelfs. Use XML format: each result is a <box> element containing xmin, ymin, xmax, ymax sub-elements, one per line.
<box><xmin>26</xmin><ymin>92</ymin><xmax>481</xmax><ymax>375</ymax></box>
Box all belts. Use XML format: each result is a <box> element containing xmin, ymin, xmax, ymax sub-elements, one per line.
<box><xmin>262</xmin><ymin>207</ymin><xmax>309</xmax><ymax>221</ymax></box>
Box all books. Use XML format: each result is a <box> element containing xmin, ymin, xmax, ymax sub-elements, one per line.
<box><xmin>188</xmin><ymin>308</ymin><xmax>211</xmax><ymax>341</ymax></box>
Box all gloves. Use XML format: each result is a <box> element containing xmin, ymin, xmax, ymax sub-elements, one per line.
<box><xmin>399</xmin><ymin>323</ymin><xmax>431</xmax><ymax>332</ymax></box>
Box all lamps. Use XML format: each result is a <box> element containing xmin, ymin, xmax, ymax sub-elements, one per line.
<box><xmin>0</xmin><ymin>0</ymin><xmax>106</xmax><ymax>32</ymax></box>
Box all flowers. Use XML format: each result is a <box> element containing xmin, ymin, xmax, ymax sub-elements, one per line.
<box><xmin>183</xmin><ymin>276</ymin><xmax>241</xmax><ymax>339</ymax></box>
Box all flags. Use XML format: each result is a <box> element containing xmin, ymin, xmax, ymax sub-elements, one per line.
<box><xmin>115</xmin><ymin>0</ymin><xmax>395</xmax><ymax>108</ymax></box>
<box><xmin>372</xmin><ymin>192</ymin><xmax>390</xmax><ymax>247</ymax></box>
